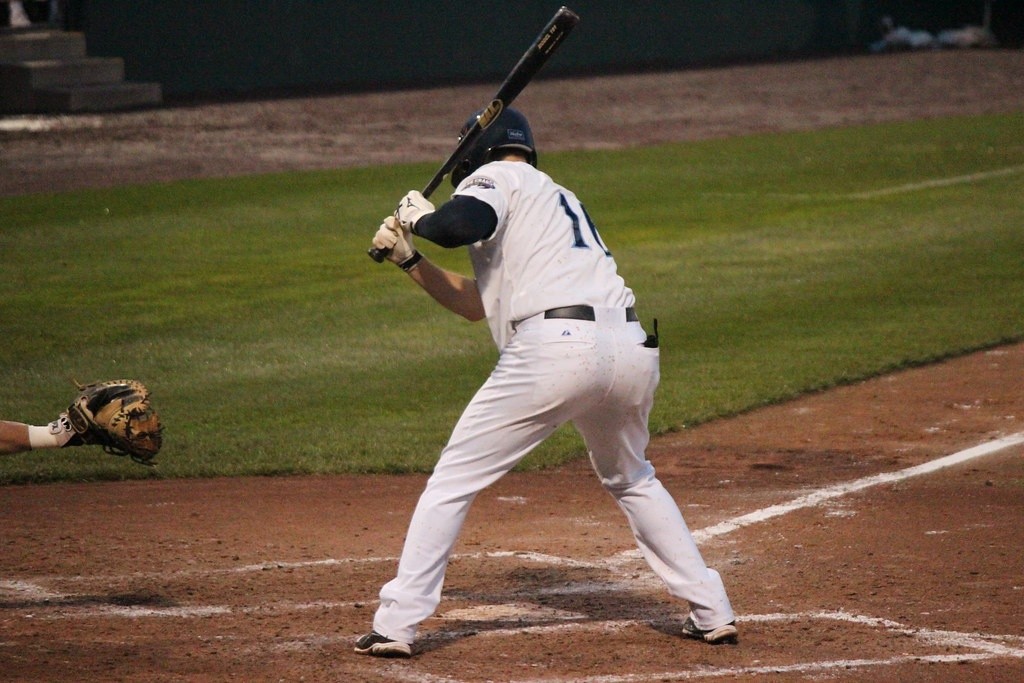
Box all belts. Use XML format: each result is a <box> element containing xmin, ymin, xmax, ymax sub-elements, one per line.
<box><xmin>545</xmin><ymin>306</ymin><xmax>638</xmax><ymax>323</ymax></box>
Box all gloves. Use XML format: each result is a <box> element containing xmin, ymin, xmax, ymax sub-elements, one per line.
<box><xmin>373</xmin><ymin>214</ymin><xmax>413</xmax><ymax>262</ymax></box>
<box><xmin>395</xmin><ymin>189</ymin><xmax>436</xmax><ymax>231</ymax></box>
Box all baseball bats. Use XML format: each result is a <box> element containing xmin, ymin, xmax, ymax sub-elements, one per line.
<box><xmin>367</xmin><ymin>8</ymin><xmax>579</xmax><ymax>263</ymax></box>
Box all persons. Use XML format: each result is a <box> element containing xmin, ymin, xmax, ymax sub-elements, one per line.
<box><xmin>0</xmin><ymin>379</ymin><xmax>160</xmax><ymax>465</ymax></box>
<box><xmin>354</xmin><ymin>106</ymin><xmax>736</xmax><ymax>658</ymax></box>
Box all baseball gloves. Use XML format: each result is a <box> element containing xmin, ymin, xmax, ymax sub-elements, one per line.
<box><xmin>63</xmin><ymin>373</ymin><xmax>162</xmax><ymax>461</ymax></box>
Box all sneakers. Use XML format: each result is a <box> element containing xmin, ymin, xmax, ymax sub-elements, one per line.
<box><xmin>682</xmin><ymin>617</ymin><xmax>739</xmax><ymax>642</ymax></box>
<box><xmin>353</xmin><ymin>632</ymin><xmax>411</xmax><ymax>657</ymax></box>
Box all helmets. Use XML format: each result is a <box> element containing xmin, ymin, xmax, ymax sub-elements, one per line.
<box><xmin>451</xmin><ymin>107</ymin><xmax>537</xmax><ymax>188</ymax></box>
